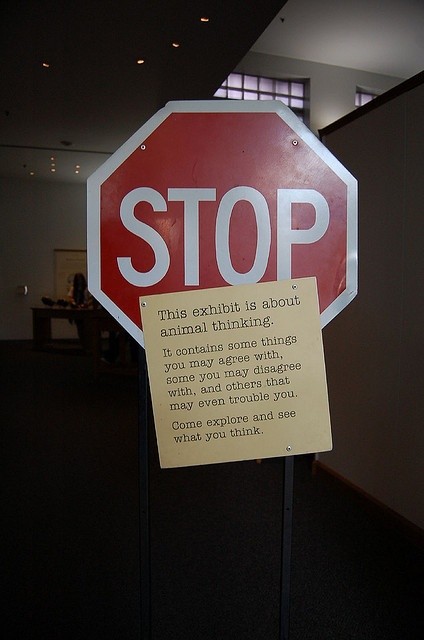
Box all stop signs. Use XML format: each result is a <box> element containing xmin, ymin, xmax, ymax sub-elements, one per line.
<box><xmin>87</xmin><ymin>101</ymin><xmax>358</xmax><ymax>349</ymax></box>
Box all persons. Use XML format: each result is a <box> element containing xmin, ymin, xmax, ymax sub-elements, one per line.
<box><xmin>66</xmin><ymin>272</ymin><xmax>90</xmax><ymax>352</ymax></box>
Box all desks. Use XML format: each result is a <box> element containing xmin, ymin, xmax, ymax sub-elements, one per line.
<box><xmin>30</xmin><ymin>308</ymin><xmax>118</xmax><ymax>384</ymax></box>
